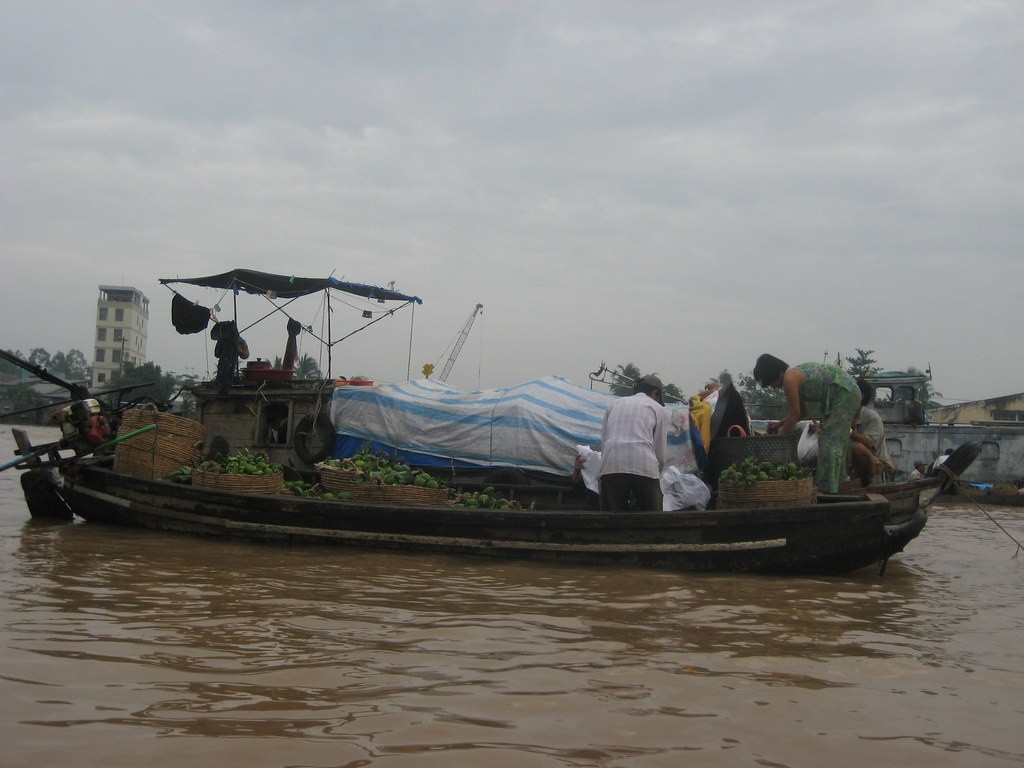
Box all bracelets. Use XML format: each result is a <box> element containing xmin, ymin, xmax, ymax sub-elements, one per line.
<box><xmin>849</xmin><ymin>427</ymin><xmax>852</xmax><ymax>437</ymax></box>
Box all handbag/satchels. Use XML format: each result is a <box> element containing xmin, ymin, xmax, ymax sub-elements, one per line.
<box><xmin>660</xmin><ymin>467</ymin><xmax>711</xmax><ymax>512</ymax></box>
<box><xmin>797</xmin><ymin>422</ymin><xmax>823</xmax><ymax>463</ymax></box>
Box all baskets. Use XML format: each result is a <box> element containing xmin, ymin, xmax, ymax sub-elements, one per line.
<box><xmin>710</xmin><ymin>425</ymin><xmax>801</xmax><ymax>471</ymax></box>
<box><xmin>349</xmin><ymin>473</ymin><xmax>449</xmax><ymax>507</ymax></box>
<box><xmin>112</xmin><ymin>404</ymin><xmax>207</xmax><ymax>482</ymax></box>
<box><xmin>716</xmin><ymin>471</ymin><xmax>814</xmax><ymax>508</ymax></box>
<box><xmin>317</xmin><ymin>461</ymin><xmax>365</xmax><ymax>494</ymax></box>
<box><xmin>191</xmin><ymin>466</ymin><xmax>282</xmax><ymax>494</ymax></box>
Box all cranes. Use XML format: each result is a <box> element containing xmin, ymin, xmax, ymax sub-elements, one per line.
<box><xmin>433</xmin><ymin>304</ymin><xmax>485</xmax><ymax>383</ymax></box>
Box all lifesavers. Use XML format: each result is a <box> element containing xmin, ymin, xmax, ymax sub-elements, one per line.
<box><xmin>294</xmin><ymin>412</ymin><xmax>336</xmax><ymax>464</ymax></box>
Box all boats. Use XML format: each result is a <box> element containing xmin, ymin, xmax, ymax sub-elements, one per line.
<box><xmin>1</xmin><ymin>266</ymin><xmax>989</xmax><ymax>583</ymax></box>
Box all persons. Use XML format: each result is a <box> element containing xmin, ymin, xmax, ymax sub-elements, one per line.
<box><xmin>569</xmin><ymin>447</ymin><xmax>601</xmax><ymax>511</ymax></box>
<box><xmin>907</xmin><ymin>460</ymin><xmax>927</xmax><ymax>481</ymax></box>
<box><xmin>811</xmin><ymin>375</ymin><xmax>895</xmax><ymax>487</ymax></box>
<box><xmin>932</xmin><ymin>448</ymin><xmax>953</xmax><ymax>475</ymax></box>
<box><xmin>755</xmin><ymin>353</ymin><xmax>863</xmax><ymax>496</ymax></box>
<box><xmin>596</xmin><ymin>374</ymin><xmax>666</xmax><ymax>513</ymax></box>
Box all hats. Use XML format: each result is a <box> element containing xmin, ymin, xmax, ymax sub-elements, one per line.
<box><xmin>914</xmin><ymin>460</ymin><xmax>927</xmax><ymax>466</ymax></box>
<box><xmin>637</xmin><ymin>374</ymin><xmax>666</xmax><ymax>408</ymax></box>
